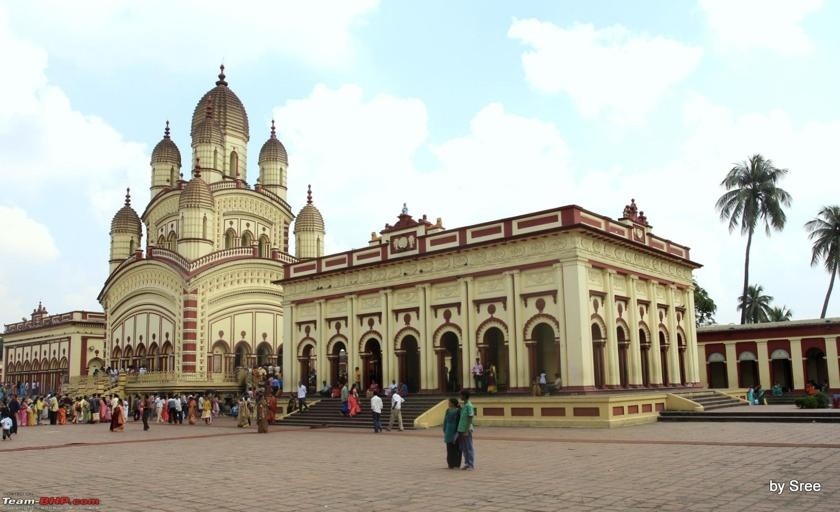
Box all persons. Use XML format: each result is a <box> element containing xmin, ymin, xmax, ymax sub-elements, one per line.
<box><xmin>532</xmin><ymin>375</ymin><xmax>541</xmax><ymax>396</ymax></box>
<box><xmin>539</xmin><ymin>369</ymin><xmax>547</xmax><ymax>395</ymax></box>
<box><xmin>472</xmin><ymin>358</ymin><xmax>484</xmax><ymax>388</ymax></box>
<box><xmin>0</xmin><ymin>412</ymin><xmax>13</xmax><ymax>441</ymax></box>
<box><xmin>0</xmin><ymin>390</ymin><xmax>267</xmax><ymax>428</ymax></box>
<box><xmin>320</xmin><ymin>380</ymin><xmax>332</xmax><ymax>397</ymax></box>
<box><xmin>255</xmin><ymin>393</ymin><xmax>270</xmax><ymax>432</ymax></box>
<box><xmin>746</xmin><ymin>378</ymin><xmax>829</xmax><ymax>406</ymax></box>
<box><xmin>546</xmin><ymin>373</ymin><xmax>561</xmax><ymax>395</ymax></box>
<box><xmin>8</xmin><ymin>393</ymin><xmax>21</xmax><ymax>435</ymax></box>
<box><xmin>0</xmin><ymin>379</ymin><xmax>40</xmax><ymax>390</ymax></box>
<box><xmin>110</xmin><ymin>398</ymin><xmax>127</xmax><ymax>432</ymax></box>
<box><xmin>330</xmin><ymin>379</ymin><xmax>344</xmax><ymax>398</ymax></box>
<box><xmin>268</xmin><ymin>386</ymin><xmax>281</xmax><ymax>425</ymax></box>
<box><xmin>244</xmin><ymin>363</ymin><xmax>282</xmax><ymax>394</ymax></box>
<box><xmin>366</xmin><ymin>380</ymin><xmax>379</xmax><ymax>399</ymax></box>
<box><xmin>385</xmin><ymin>389</ymin><xmax>406</xmax><ymax>431</ymax></box>
<box><xmin>354</xmin><ymin>367</ymin><xmax>361</xmax><ymax>396</ymax></box>
<box><xmin>398</xmin><ymin>382</ymin><xmax>409</xmax><ymax>397</ymax></box>
<box><xmin>443</xmin><ymin>398</ymin><xmax>463</xmax><ymax>468</ymax></box>
<box><xmin>348</xmin><ymin>385</ymin><xmax>361</xmax><ymax>417</ymax></box>
<box><xmin>142</xmin><ymin>393</ymin><xmax>152</xmax><ymax>431</ymax></box>
<box><xmin>485</xmin><ymin>360</ymin><xmax>497</xmax><ymax>388</ymax></box>
<box><xmin>93</xmin><ymin>364</ymin><xmax>148</xmax><ymax>380</ymax></box>
<box><xmin>482</xmin><ymin>368</ymin><xmax>495</xmax><ymax>391</ymax></box>
<box><xmin>371</xmin><ymin>391</ymin><xmax>384</xmax><ymax>433</ymax></box>
<box><xmin>385</xmin><ymin>379</ymin><xmax>397</xmax><ymax>400</ymax></box>
<box><xmin>338</xmin><ymin>380</ymin><xmax>349</xmax><ymax>416</ymax></box>
<box><xmin>297</xmin><ymin>381</ymin><xmax>311</xmax><ymax>414</ymax></box>
<box><xmin>458</xmin><ymin>389</ymin><xmax>475</xmax><ymax>471</ymax></box>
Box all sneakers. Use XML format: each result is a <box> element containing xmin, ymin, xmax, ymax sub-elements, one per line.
<box><xmin>448</xmin><ymin>465</ymin><xmax>475</xmax><ymax>470</ymax></box>
